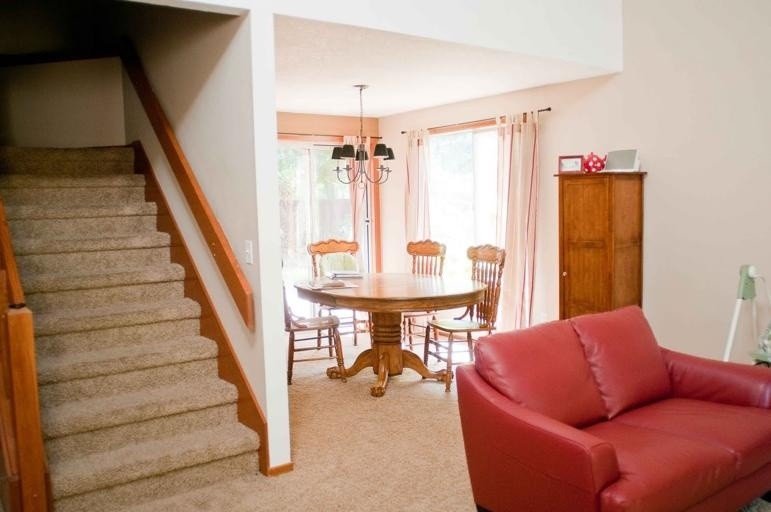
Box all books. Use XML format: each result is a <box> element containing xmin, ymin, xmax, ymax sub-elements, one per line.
<box><xmin>327</xmin><ymin>271</ymin><xmax>362</xmax><ymax>280</ymax></box>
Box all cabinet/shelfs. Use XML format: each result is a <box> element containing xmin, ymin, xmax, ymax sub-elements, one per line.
<box><xmin>553</xmin><ymin>172</ymin><xmax>646</xmax><ymax>323</ymax></box>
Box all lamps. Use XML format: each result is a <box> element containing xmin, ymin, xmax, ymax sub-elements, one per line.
<box><xmin>331</xmin><ymin>144</ymin><xmax>395</xmax><ymax>184</ymax></box>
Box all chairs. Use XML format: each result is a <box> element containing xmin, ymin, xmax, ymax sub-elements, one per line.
<box><xmin>309</xmin><ymin>240</ymin><xmax>359</xmax><ymax>346</ymax></box>
<box><xmin>423</xmin><ymin>242</ymin><xmax>506</xmax><ymax>392</ymax></box>
<box><xmin>284</xmin><ymin>280</ymin><xmax>347</xmax><ymax>384</ymax></box>
<box><xmin>401</xmin><ymin>240</ymin><xmax>445</xmax><ymax>352</ymax></box>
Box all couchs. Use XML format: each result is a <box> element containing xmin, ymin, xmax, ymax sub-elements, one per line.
<box><xmin>456</xmin><ymin>306</ymin><xmax>771</xmax><ymax>511</ymax></box>
